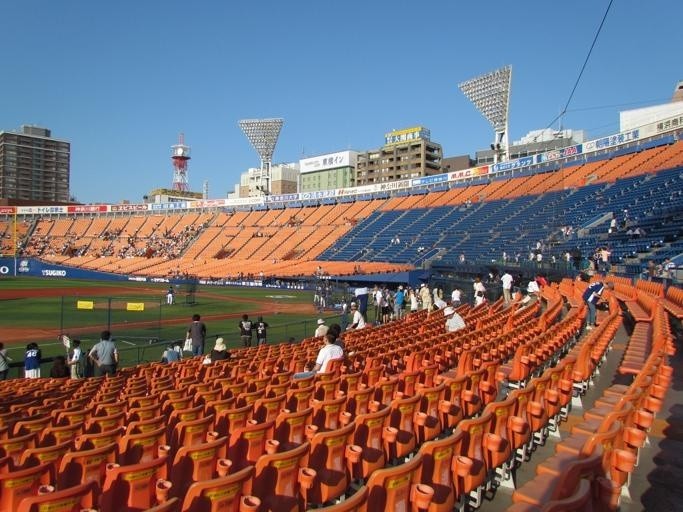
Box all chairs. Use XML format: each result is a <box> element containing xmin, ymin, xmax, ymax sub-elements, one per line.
<box><xmin>1</xmin><ymin>138</ymin><xmax>683</xmax><ymax>511</ymax></box>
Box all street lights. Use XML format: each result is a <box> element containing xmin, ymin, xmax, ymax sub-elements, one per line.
<box><xmin>236</xmin><ymin>117</ymin><xmax>285</xmax><ymax>195</ymax></box>
<box><xmin>457</xmin><ymin>62</ymin><xmax>512</xmax><ymax>164</ymax></box>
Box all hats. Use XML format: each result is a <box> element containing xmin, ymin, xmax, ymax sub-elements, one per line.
<box><xmin>444</xmin><ymin>307</ymin><xmax>454</xmax><ymax>316</ymax></box>
<box><xmin>214</xmin><ymin>338</ymin><xmax>226</xmax><ymax>352</ymax></box>
<box><xmin>318</xmin><ymin>319</ymin><xmax>325</xmax><ymax>324</ymax></box>
